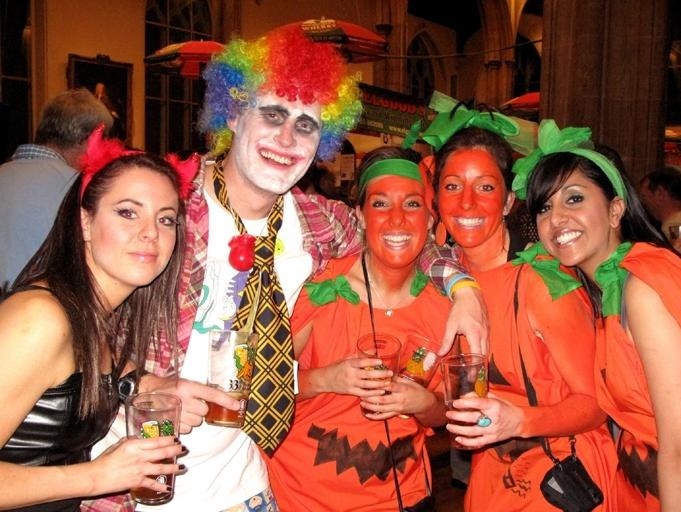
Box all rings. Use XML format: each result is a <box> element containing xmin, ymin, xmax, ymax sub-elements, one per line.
<box><xmin>477</xmin><ymin>412</ymin><xmax>492</xmax><ymax>428</ymax></box>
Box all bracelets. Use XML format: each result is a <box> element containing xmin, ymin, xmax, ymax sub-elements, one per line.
<box><xmin>450</xmin><ymin>281</ymin><xmax>481</xmax><ymax>296</ymax></box>
<box><xmin>446</xmin><ymin>273</ymin><xmax>475</xmax><ymax>301</ymax></box>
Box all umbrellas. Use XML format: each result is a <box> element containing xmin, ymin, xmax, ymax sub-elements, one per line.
<box><xmin>290</xmin><ymin>15</ymin><xmax>389</xmax><ymax>64</ymax></box>
<box><xmin>499</xmin><ymin>91</ymin><xmax>540</xmax><ymax>111</ymax></box>
<box><xmin>144</xmin><ymin>38</ymin><xmax>226</xmax><ymax>81</ymax></box>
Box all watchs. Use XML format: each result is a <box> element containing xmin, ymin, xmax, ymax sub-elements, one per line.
<box><xmin>118</xmin><ymin>367</ymin><xmax>148</xmax><ymax>406</ymax></box>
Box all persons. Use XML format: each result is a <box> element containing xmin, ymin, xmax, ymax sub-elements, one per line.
<box><xmin>640</xmin><ymin>167</ymin><xmax>681</xmax><ymax>260</ymax></box>
<box><xmin>90</xmin><ymin>25</ymin><xmax>491</xmax><ymax>512</ymax></box>
<box><xmin>0</xmin><ymin>87</ymin><xmax>114</xmax><ymax>303</ymax></box>
<box><xmin>0</xmin><ymin>124</ymin><xmax>186</xmax><ymax>512</ymax></box>
<box><xmin>258</xmin><ymin>147</ymin><xmax>461</xmax><ymax>512</ymax></box>
<box><xmin>93</xmin><ymin>82</ymin><xmax>119</xmax><ymax>119</ymax></box>
<box><xmin>434</xmin><ymin>127</ymin><xmax>619</xmax><ymax>512</ymax></box>
<box><xmin>509</xmin><ymin>118</ymin><xmax>681</xmax><ymax>512</ymax></box>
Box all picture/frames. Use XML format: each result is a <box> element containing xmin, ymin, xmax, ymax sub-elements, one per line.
<box><xmin>67</xmin><ymin>53</ymin><xmax>134</xmax><ymax>150</ymax></box>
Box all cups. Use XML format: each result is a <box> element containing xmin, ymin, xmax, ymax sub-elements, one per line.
<box><xmin>205</xmin><ymin>329</ymin><xmax>260</xmax><ymax>428</ymax></box>
<box><xmin>396</xmin><ymin>333</ymin><xmax>441</xmax><ymax>420</ymax></box>
<box><xmin>125</xmin><ymin>392</ymin><xmax>182</xmax><ymax>507</ymax></box>
<box><xmin>441</xmin><ymin>354</ymin><xmax>489</xmax><ymax>449</ymax></box>
<box><xmin>356</xmin><ymin>331</ymin><xmax>403</xmax><ymax>418</ymax></box>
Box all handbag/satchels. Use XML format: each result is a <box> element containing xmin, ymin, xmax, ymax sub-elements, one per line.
<box><xmin>540</xmin><ymin>455</ymin><xmax>603</xmax><ymax>512</ymax></box>
